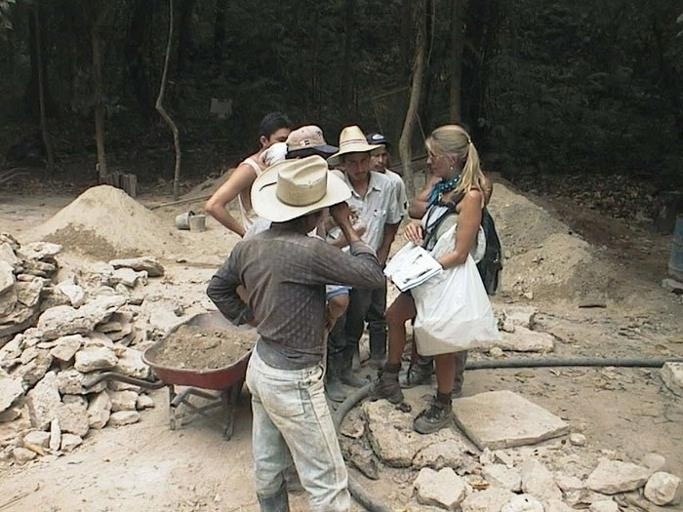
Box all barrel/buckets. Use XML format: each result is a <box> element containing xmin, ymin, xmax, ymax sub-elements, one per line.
<box><xmin>667</xmin><ymin>213</ymin><xmax>683</xmax><ymax>282</ymax></box>
<box><xmin>189</xmin><ymin>213</ymin><xmax>207</xmax><ymax>234</ymax></box>
<box><xmin>175</xmin><ymin>210</ymin><xmax>196</xmax><ymax>229</ymax></box>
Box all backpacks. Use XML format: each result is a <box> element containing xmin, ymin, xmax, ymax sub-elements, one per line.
<box><xmin>434</xmin><ymin>185</ymin><xmax>502</xmax><ymax>295</ymax></box>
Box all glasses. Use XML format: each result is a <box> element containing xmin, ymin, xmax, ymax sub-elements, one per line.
<box><xmin>430</xmin><ymin>153</ymin><xmax>439</xmax><ymax>161</ymax></box>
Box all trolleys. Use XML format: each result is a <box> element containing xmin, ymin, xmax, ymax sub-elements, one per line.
<box><xmin>81</xmin><ymin>311</ymin><xmax>257</xmax><ymax>442</ymax></box>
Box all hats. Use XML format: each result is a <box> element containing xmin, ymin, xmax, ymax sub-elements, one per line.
<box><xmin>327</xmin><ymin>126</ymin><xmax>389</xmax><ymax>165</ymax></box>
<box><xmin>251</xmin><ymin>125</ymin><xmax>352</xmax><ymax>222</ymax></box>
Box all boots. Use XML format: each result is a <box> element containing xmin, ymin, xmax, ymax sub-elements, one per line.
<box><xmin>399</xmin><ymin>355</ymin><xmax>432</xmax><ymax>388</ymax></box>
<box><xmin>368</xmin><ymin>378</ymin><xmax>403</xmax><ymax>403</ymax></box>
<box><xmin>256</xmin><ymin>479</ymin><xmax>289</xmax><ymax>512</ymax></box>
<box><xmin>360</xmin><ymin>333</ymin><xmax>387</xmax><ymax>370</ymax></box>
<box><xmin>327</xmin><ymin>368</ymin><xmax>364</xmax><ymax>402</ymax></box>
<box><xmin>414</xmin><ymin>397</ymin><xmax>453</xmax><ymax>434</ymax></box>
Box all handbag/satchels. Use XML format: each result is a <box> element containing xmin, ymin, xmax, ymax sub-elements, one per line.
<box><xmin>411</xmin><ymin>253</ymin><xmax>503</xmax><ymax>356</ymax></box>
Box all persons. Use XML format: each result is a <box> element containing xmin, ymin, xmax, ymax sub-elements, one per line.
<box><xmin>202</xmin><ymin>155</ymin><xmax>386</xmax><ymax>511</ymax></box>
